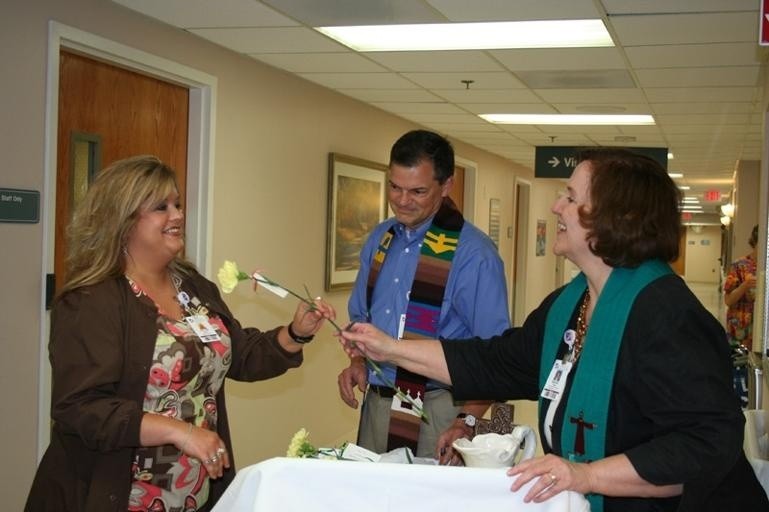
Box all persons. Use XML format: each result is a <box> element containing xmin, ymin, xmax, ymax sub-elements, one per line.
<box><xmin>328</xmin><ymin>147</ymin><xmax>768</xmax><ymax>511</ymax></box>
<box><xmin>20</xmin><ymin>153</ymin><xmax>337</xmax><ymax>512</ymax></box>
<box><xmin>723</xmin><ymin>224</ymin><xmax>759</xmax><ymax>408</ymax></box>
<box><xmin>335</xmin><ymin>129</ymin><xmax>513</xmax><ymax>466</ymax></box>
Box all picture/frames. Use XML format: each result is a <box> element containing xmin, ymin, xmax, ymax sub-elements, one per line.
<box><xmin>324</xmin><ymin>151</ymin><xmax>390</xmax><ymax>295</ymax></box>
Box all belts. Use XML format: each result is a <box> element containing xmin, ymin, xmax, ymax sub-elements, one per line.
<box><xmin>368</xmin><ymin>381</ymin><xmax>440</xmax><ymax>395</ymax></box>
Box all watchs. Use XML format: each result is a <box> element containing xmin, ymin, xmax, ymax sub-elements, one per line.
<box><xmin>455</xmin><ymin>413</ymin><xmax>475</xmax><ymax>427</ymax></box>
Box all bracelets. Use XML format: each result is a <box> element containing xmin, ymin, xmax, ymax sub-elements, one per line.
<box><xmin>287</xmin><ymin>322</ymin><xmax>315</xmax><ymax>344</ymax></box>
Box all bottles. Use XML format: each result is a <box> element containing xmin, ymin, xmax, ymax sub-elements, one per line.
<box><xmin>452</xmin><ymin>425</ymin><xmax>537</xmax><ymax>469</ymax></box>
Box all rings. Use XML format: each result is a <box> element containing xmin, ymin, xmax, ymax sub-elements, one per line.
<box><xmin>210</xmin><ymin>456</ymin><xmax>217</xmax><ymax>462</ymax></box>
<box><xmin>548</xmin><ymin>474</ymin><xmax>556</xmax><ymax>482</ymax></box>
<box><xmin>204</xmin><ymin>459</ymin><xmax>211</xmax><ymax>465</ymax></box>
<box><xmin>215</xmin><ymin>448</ymin><xmax>224</xmax><ymax>458</ymax></box>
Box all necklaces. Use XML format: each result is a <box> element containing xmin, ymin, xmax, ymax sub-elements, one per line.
<box><xmin>573</xmin><ymin>288</ymin><xmax>588</xmax><ymax>356</ymax></box>
